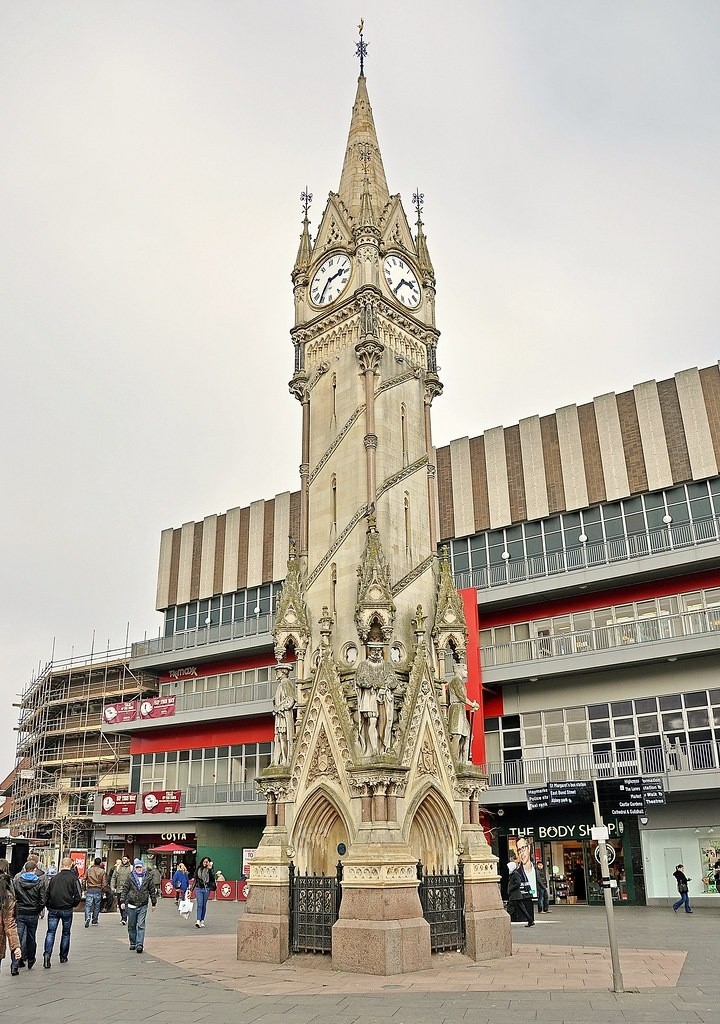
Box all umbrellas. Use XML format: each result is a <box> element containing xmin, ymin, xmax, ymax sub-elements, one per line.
<box><xmin>147</xmin><ymin>842</ymin><xmax>195</xmax><ymax>879</ymax></box>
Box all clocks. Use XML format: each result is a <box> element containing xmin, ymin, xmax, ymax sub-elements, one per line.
<box><xmin>380</xmin><ymin>249</ymin><xmax>423</xmax><ymax>313</ymax></box>
<box><xmin>306</xmin><ymin>250</ymin><xmax>356</xmax><ymax>312</ymax></box>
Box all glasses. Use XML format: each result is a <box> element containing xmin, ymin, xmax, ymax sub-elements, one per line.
<box><xmin>136</xmin><ymin>866</ymin><xmax>141</xmax><ymax>868</ymax></box>
<box><xmin>517</xmin><ymin>845</ymin><xmax>528</xmax><ymax>853</ymax></box>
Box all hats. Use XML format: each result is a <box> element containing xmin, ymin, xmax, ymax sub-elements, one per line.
<box><xmin>26</xmin><ymin>861</ymin><xmax>37</xmax><ymax>868</ymax></box>
<box><xmin>134</xmin><ymin>858</ymin><xmax>143</xmax><ymax>868</ymax></box>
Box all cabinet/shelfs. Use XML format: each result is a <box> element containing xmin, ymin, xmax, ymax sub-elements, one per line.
<box><xmin>549</xmin><ymin>875</ymin><xmax>570</xmax><ymax>899</ymax></box>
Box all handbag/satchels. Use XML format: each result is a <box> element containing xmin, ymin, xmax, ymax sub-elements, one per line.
<box><xmin>178</xmin><ymin>897</ymin><xmax>193</xmax><ymax>919</ymax></box>
<box><xmin>177</xmin><ymin>881</ymin><xmax>181</xmax><ymax>887</ymax></box>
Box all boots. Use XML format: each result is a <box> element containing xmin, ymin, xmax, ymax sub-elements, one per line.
<box><xmin>194</xmin><ymin>920</ymin><xmax>205</xmax><ymax>928</ymax></box>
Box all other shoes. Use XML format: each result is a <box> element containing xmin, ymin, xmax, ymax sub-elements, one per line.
<box><xmin>85</xmin><ymin>918</ymin><xmax>90</xmax><ymax>928</ymax></box>
<box><xmin>60</xmin><ymin>957</ymin><xmax>68</xmax><ymax>963</ymax></box>
<box><xmin>11</xmin><ymin>972</ymin><xmax>19</xmax><ymax>976</ymax></box>
<box><xmin>130</xmin><ymin>945</ymin><xmax>135</xmax><ymax>950</ymax></box>
<box><xmin>525</xmin><ymin>922</ymin><xmax>536</xmax><ymax>928</ymax></box>
<box><xmin>137</xmin><ymin>948</ymin><xmax>143</xmax><ymax>953</ymax></box>
<box><xmin>122</xmin><ymin>920</ymin><xmax>127</xmax><ymax>925</ymax></box>
<box><xmin>44</xmin><ymin>954</ymin><xmax>51</xmax><ymax>968</ymax></box>
<box><xmin>541</xmin><ymin>910</ymin><xmax>553</xmax><ymax>914</ymax></box>
<box><xmin>101</xmin><ymin>908</ymin><xmax>108</xmax><ymax>913</ymax></box>
<box><xmin>92</xmin><ymin>921</ymin><xmax>98</xmax><ymax>925</ymax></box>
<box><xmin>686</xmin><ymin>911</ymin><xmax>693</xmax><ymax>913</ymax></box>
<box><xmin>674</xmin><ymin>909</ymin><xmax>677</xmax><ymax>913</ymax></box>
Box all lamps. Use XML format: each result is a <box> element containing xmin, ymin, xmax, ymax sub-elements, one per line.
<box><xmin>668</xmin><ymin>657</ymin><xmax>677</xmax><ymax>661</ymax></box>
<box><xmin>529</xmin><ymin>678</ymin><xmax>539</xmax><ymax>681</ymax></box>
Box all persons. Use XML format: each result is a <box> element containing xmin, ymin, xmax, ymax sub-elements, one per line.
<box><xmin>84</xmin><ymin>855</ymin><xmax>161</xmax><ymax>928</ymax></box>
<box><xmin>672</xmin><ymin>864</ymin><xmax>693</xmax><ymax>913</ymax></box>
<box><xmin>536</xmin><ymin>862</ymin><xmax>552</xmax><ymax>914</ymax></box>
<box><xmin>516</xmin><ymin>836</ymin><xmax>537</xmax><ymax>897</ymax></box>
<box><xmin>448</xmin><ymin>664</ymin><xmax>480</xmax><ymax>765</ymax></box>
<box><xmin>171</xmin><ymin>863</ymin><xmax>189</xmax><ymax>904</ymax></box>
<box><xmin>507</xmin><ymin>862</ymin><xmax>535</xmax><ymax>927</ymax></box>
<box><xmin>187</xmin><ymin>856</ymin><xmax>217</xmax><ymax>928</ymax></box>
<box><xmin>0</xmin><ymin>853</ymin><xmax>82</xmax><ymax>975</ymax></box>
<box><xmin>120</xmin><ymin>861</ymin><xmax>157</xmax><ymax>953</ymax></box>
<box><xmin>267</xmin><ymin>663</ymin><xmax>297</xmax><ymax>767</ymax></box>
<box><xmin>355</xmin><ymin>642</ymin><xmax>406</xmax><ymax>758</ymax></box>
<box><xmin>571</xmin><ymin>863</ymin><xmax>586</xmax><ymax>900</ymax></box>
<box><xmin>215</xmin><ymin>870</ymin><xmax>225</xmax><ymax>881</ymax></box>
